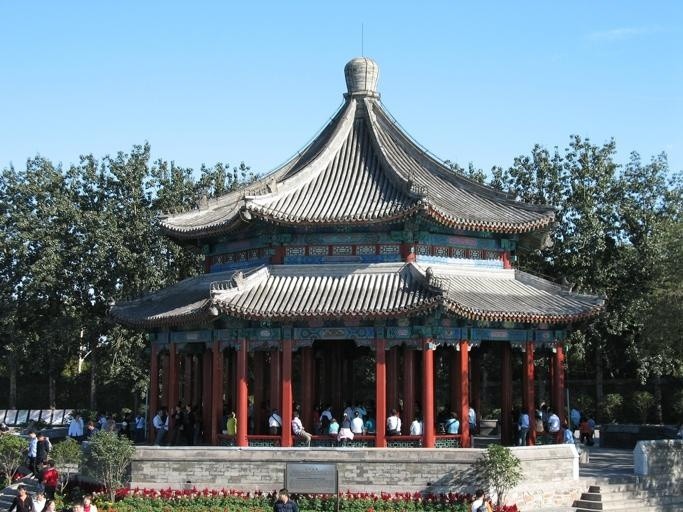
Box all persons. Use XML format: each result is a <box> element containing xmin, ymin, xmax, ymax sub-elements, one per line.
<box><xmin>408</xmin><ymin>405</ymin><xmax>477</xmax><ymax>436</ymax></box>
<box><xmin>314</xmin><ymin>403</ymin><xmax>402</xmax><ymax>442</ymax></box>
<box><xmin>467</xmin><ymin>489</ymin><xmax>492</xmax><ymax>512</ymax></box>
<box><xmin>221</xmin><ymin>402</ymin><xmax>312</xmax><ymax>447</ymax></box>
<box><xmin>68</xmin><ymin>404</ymin><xmax>197</xmax><ymax>446</ymax></box>
<box><xmin>509</xmin><ymin>403</ymin><xmax>594</xmax><ymax>447</ymax></box>
<box><xmin>6</xmin><ymin>430</ymin><xmax>97</xmax><ymax>511</ymax></box>
<box><xmin>272</xmin><ymin>487</ymin><xmax>299</xmax><ymax>512</ymax></box>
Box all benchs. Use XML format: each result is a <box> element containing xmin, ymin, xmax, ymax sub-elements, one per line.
<box><xmin>214</xmin><ymin>432</ymin><xmax>462</xmax><ymax>449</ymax></box>
